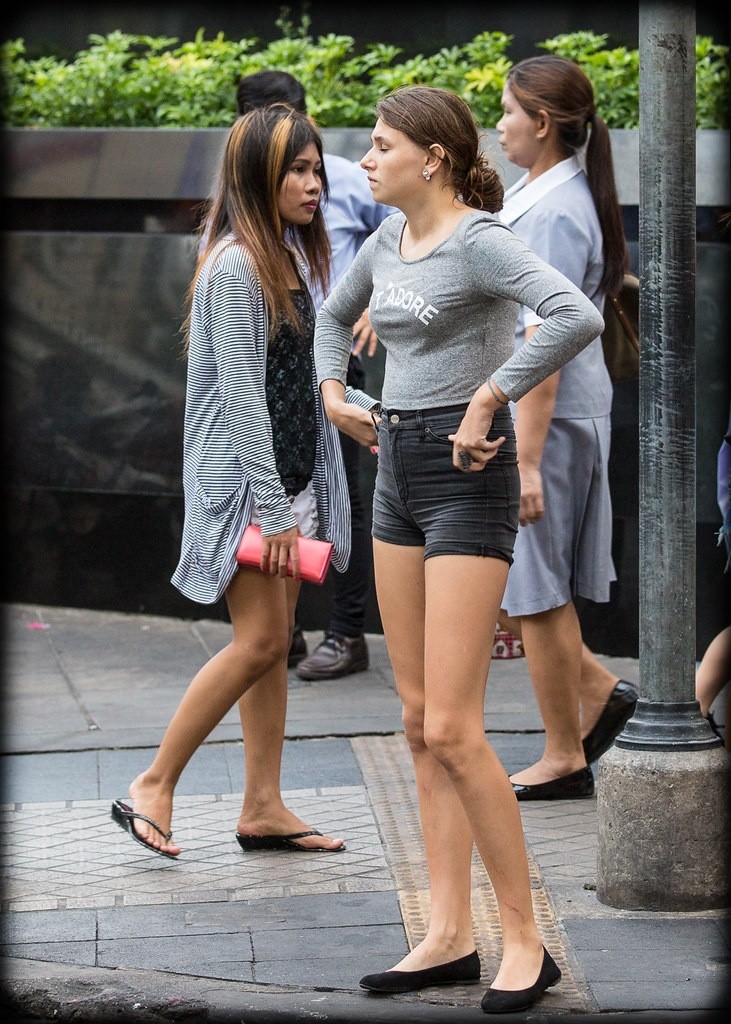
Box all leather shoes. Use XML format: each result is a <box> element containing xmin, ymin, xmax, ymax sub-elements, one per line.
<box><xmin>582</xmin><ymin>679</ymin><xmax>639</xmax><ymax>765</ymax></box>
<box><xmin>504</xmin><ymin>766</ymin><xmax>595</xmax><ymax>800</ymax></box>
<box><xmin>289</xmin><ymin>630</ymin><xmax>307</xmax><ymax>668</ymax></box>
<box><xmin>296</xmin><ymin>634</ymin><xmax>370</xmax><ymax>677</ymax></box>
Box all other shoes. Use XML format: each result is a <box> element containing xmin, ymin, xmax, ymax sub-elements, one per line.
<box><xmin>482</xmin><ymin>944</ymin><xmax>561</xmax><ymax>1012</ymax></box>
<box><xmin>358</xmin><ymin>948</ymin><xmax>481</xmax><ymax>992</ymax></box>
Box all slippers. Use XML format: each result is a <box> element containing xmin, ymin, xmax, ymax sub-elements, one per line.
<box><xmin>235</xmin><ymin>830</ymin><xmax>347</xmax><ymax>852</ymax></box>
<box><xmin>111</xmin><ymin>800</ymin><xmax>179</xmax><ymax>861</ymax></box>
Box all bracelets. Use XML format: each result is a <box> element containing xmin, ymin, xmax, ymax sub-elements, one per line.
<box><xmin>487</xmin><ymin>379</ymin><xmax>509</xmax><ymax>406</ymax></box>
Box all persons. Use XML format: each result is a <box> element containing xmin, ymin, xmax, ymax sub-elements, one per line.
<box><xmin>233</xmin><ymin>70</ymin><xmax>389</xmax><ymax>683</ymax></box>
<box><xmin>690</xmin><ymin>410</ymin><xmax>731</xmax><ymax>734</ymax></box>
<box><xmin>110</xmin><ymin>101</ymin><xmax>346</xmax><ymax>858</ymax></box>
<box><xmin>314</xmin><ymin>83</ymin><xmax>607</xmax><ymax>1016</ymax></box>
<box><xmin>490</xmin><ymin>54</ymin><xmax>639</xmax><ymax>804</ymax></box>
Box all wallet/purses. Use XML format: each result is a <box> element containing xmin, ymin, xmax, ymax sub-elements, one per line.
<box><xmin>237</xmin><ymin>525</ymin><xmax>335</xmax><ymax>585</ymax></box>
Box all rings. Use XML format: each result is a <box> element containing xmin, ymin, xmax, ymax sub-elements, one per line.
<box><xmin>457</xmin><ymin>450</ymin><xmax>473</xmax><ymax>470</ymax></box>
